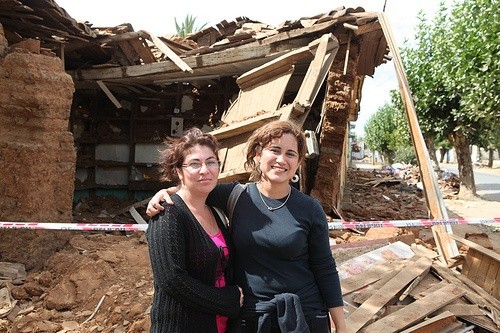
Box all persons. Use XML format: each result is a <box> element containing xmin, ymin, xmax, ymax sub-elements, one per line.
<box><xmin>145</xmin><ymin>120</ymin><xmax>348</xmax><ymax>333</ymax></box>
<box><xmin>144</xmin><ymin>126</ymin><xmax>246</xmax><ymax>333</ymax></box>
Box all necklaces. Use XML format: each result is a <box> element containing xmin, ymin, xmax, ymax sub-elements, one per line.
<box><xmin>257</xmin><ymin>182</ymin><xmax>292</xmax><ymax>210</ymax></box>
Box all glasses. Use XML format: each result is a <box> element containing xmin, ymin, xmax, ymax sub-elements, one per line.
<box><xmin>178</xmin><ymin>160</ymin><xmax>222</xmax><ymax>169</ymax></box>
